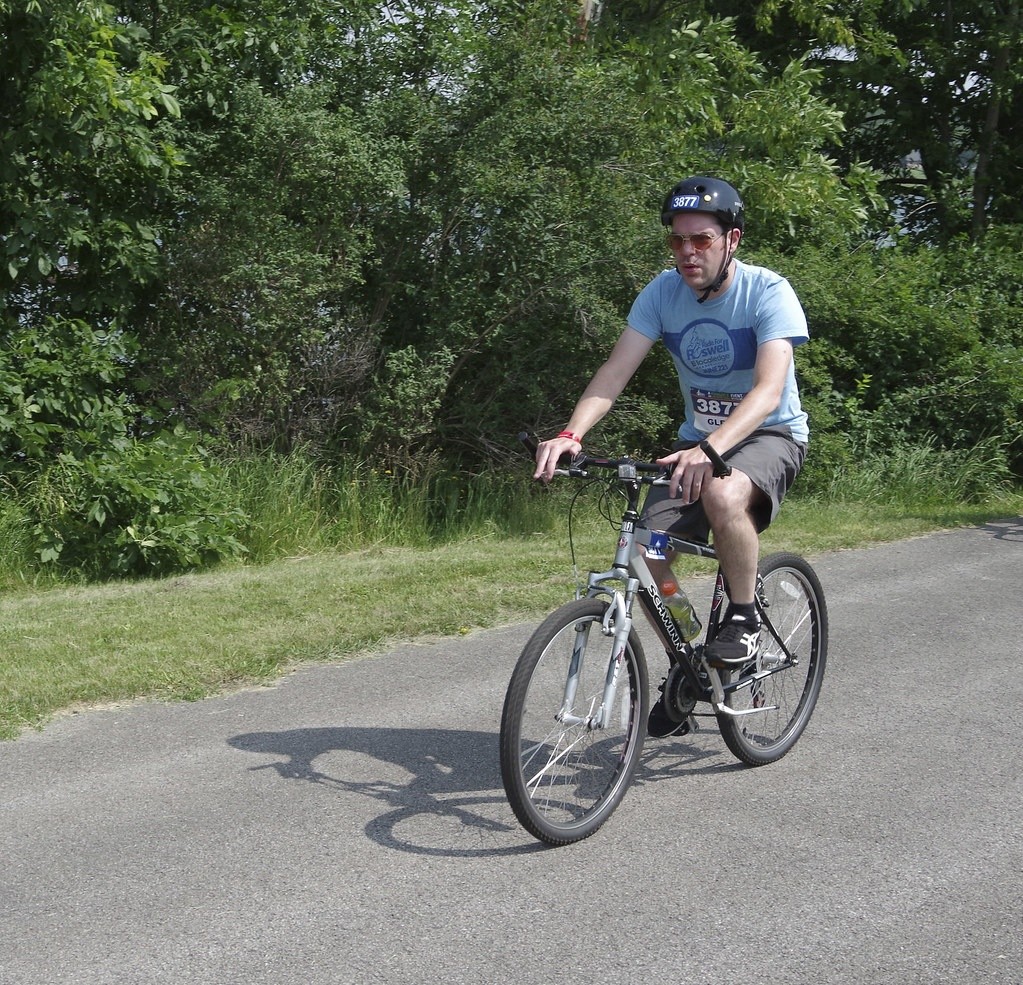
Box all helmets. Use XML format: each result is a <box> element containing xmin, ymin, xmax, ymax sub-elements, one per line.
<box><xmin>661</xmin><ymin>176</ymin><xmax>745</xmax><ymax>233</ymax></box>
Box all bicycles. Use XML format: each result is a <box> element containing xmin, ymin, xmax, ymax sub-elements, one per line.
<box><xmin>499</xmin><ymin>430</ymin><xmax>829</xmax><ymax>847</ymax></box>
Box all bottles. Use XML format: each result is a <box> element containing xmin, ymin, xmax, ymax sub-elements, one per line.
<box><xmin>659</xmin><ymin>580</ymin><xmax>700</xmax><ymax>642</ymax></box>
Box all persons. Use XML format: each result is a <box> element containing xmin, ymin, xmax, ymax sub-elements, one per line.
<box><xmin>533</xmin><ymin>177</ymin><xmax>809</xmax><ymax>738</ymax></box>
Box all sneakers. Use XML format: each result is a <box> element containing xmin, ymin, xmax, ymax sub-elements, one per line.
<box><xmin>646</xmin><ymin>668</ymin><xmax>690</xmax><ymax>738</ymax></box>
<box><xmin>704</xmin><ymin>610</ymin><xmax>762</xmax><ymax>664</ymax></box>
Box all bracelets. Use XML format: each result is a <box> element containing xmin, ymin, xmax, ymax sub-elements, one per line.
<box><xmin>556</xmin><ymin>432</ymin><xmax>580</xmax><ymax>443</ymax></box>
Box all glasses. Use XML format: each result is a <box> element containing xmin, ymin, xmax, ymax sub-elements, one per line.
<box><xmin>665</xmin><ymin>232</ymin><xmax>727</xmax><ymax>251</ymax></box>
